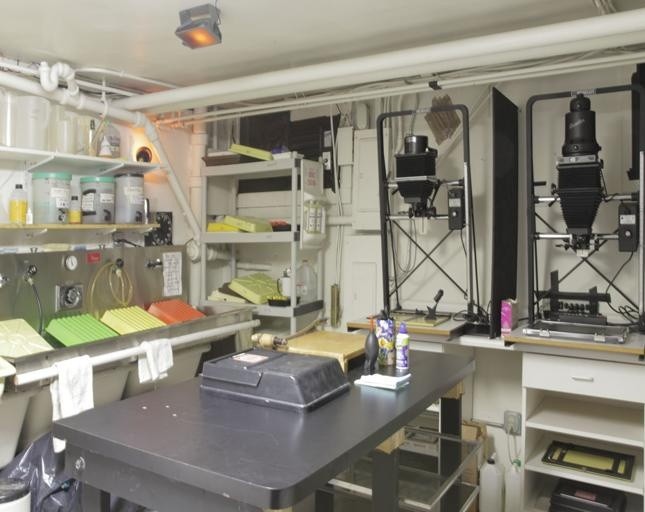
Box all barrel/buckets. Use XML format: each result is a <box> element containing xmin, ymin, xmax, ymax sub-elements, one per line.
<box><xmin>478</xmin><ymin>450</ymin><xmax>505</xmax><ymax>512</ymax></box>
<box><xmin>79</xmin><ymin>177</ymin><xmax>116</xmax><ymax>225</ymax></box>
<box><xmin>296</xmin><ymin>258</ymin><xmax>318</xmax><ymax>304</ymax></box>
<box><xmin>32</xmin><ymin>171</ymin><xmax>72</xmax><ymax>224</ymax></box>
<box><xmin>114</xmin><ymin>173</ymin><xmax>145</xmax><ymax>223</ymax></box>
<box><xmin>505</xmin><ymin>459</ymin><xmax>520</xmax><ymax>512</ymax></box>
<box><xmin>96</xmin><ymin>118</ymin><xmax>121</xmax><ymax>157</ymax></box>
<box><xmin>16</xmin><ymin>94</ymin><xmax>52</xmax><ymax>149</ymax></box>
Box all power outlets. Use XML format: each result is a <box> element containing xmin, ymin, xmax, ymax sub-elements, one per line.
<box><xmin>504</xmin><ymin>410</ymin><xmax>522</xmax><ymax>436</ymax></box>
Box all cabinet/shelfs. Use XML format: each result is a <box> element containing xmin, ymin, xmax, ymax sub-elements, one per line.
<box><xmin>1</xmin><ymin>146</ymin><xmax>160</xmax><ymax>239</ymax></box>
<box><xmin>51</xmin><ymin>348</ymin><xmax>475</xmax><ymax>511</ymax></box>
<box><xmin>198</xmin><ymin>151</ymin><xmax>328</xmax><ymax>338</ymax></box>
<box><xmin>518</xmin><ymin>352</ymin><xmax>644</xmax><ymax>511</ymax></box>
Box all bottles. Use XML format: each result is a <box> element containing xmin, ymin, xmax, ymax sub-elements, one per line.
<box><xmin>9</xmin><ymin>183</ymin><xmax>28</xmax><ymax>222</ymax></box>
<box><xmin>88</xmin><ymin>117</ymin><xmax>98</xmax><ymax>157</ymax></box>
<box><xmin>68</xmin><ymin>195</ymin><xmax>80</xmax><ymax>223</ymax></box>
<box><xmin>396</xmin><ymin>322</ymin><xmax>409</xmax><ymax>371</ymax></box>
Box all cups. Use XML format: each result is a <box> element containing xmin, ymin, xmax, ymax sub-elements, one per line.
<box><xmin>277</xmin><ymin>276</ymin><xmax>291</xmax><ymax>296</ymax></box>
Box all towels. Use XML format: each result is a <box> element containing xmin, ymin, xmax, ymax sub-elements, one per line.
<box><xmin>137</xmin><ymin>339</ymin><xmax>173</xmax><ymax>383</ymax></box>
<box><xmin>355</xmin><ymin>373</ymin><xmax>412</xmax><ymax>390</ymax></box>
<box><xmin>49</xmin><ymin>354</ymin><xmax>95</xmax><ymax>453</ymax></box>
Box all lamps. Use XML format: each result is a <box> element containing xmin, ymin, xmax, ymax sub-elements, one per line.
<box><xmin>175</xmin><ymin>5</ymin><xmax>221</xmax><ymax>50</ymax></box>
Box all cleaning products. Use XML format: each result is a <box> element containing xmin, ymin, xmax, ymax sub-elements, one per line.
<box><xmin>363</xmin><ymin>314</ymin><xmax>382</xmax><ymax>375</ymax></box>
